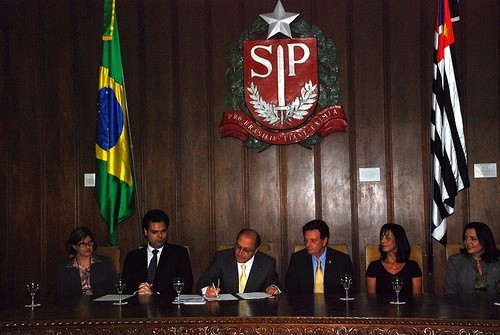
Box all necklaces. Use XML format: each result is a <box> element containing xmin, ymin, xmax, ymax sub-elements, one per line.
<box><xmin>394</xmin><ymin>267</ymin><xmax>396</xmax><ymax>269</ymax></box>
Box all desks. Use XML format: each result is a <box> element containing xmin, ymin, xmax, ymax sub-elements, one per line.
<box><xmin>0</xmin><ymin>295</ymin><xmax>500</xmax><ymax>335</ymax></box>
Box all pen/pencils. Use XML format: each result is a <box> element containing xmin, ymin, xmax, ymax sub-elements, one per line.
<box><xmin>212</xmin><ymin>282</ymin><xmax>219</xmax><ymax>297</ymax></box>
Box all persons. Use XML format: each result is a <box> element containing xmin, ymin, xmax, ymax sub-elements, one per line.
<box><xmin>122</xmin><ymin>209</ymin><xmax>193</xmax><ymax>295</ymax></box>
<box><xmin>285</xmin><ymin>220</ymin><xmax>355</xmax><ymax>294</ymax></box>
<box><xmin>59</xmin><ymin>226</ymin><xmax>116</xmax><ymax>299</ymax></box>
<box><xmin>442</xmin><ymin>222</ymin><xmax>500</xmax><ymax>295</ymax></box>
<box><xmin>195</xmin><ymin>229</ymin><xmax>281</xmax><ymax>298</ymax></box>
<box><xmin>365</xmin><ymin>223</ymin><xmax>422</xmax><ymax>294</ymax></box>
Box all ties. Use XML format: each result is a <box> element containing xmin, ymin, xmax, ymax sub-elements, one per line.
<box><xmin>315</xmin><ymin>259</ymin><xmax>324</xmax><ymax>293</ymax></box>
<box><xmin>239</xmin><ymin>265</ymin><xmax>247</xmax><ymax>293</ymax></box>
<box><xmin>147</xmin><ymin>250</ymin><xmax>159</xmax><ymax>283</ymax></box>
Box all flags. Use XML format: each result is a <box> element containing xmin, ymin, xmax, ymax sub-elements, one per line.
<box><xmin>94</xmin><ymin>0</ymin><xmax>136</xmax><ymax>244</ymax></box>
<box><xmin>431</xmin><ymin>0</ymin><xmax>471</xmax><ymax>246</ymax></box>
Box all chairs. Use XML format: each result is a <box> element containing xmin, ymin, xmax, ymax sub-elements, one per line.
<box><xmin>96</xmin><ymin>246</ymin><xmax>120</xmax><ymax>273</ymax></box>
<box><xmin>365</xmin><ymin>245</ymin><xmax>421</xmax><ymax>270</ymax></box>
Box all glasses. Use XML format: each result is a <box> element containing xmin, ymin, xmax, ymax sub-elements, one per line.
<box><xmin>76</xmin><ymin>242</ymin><xmax>95</xmax><ymax>248</ymax></box>
